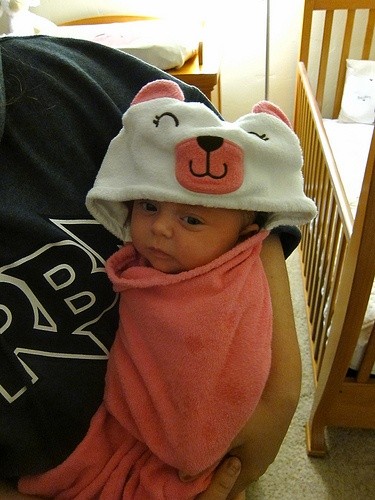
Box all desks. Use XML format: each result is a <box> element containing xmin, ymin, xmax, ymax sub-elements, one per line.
<box><xmin>53</xmin><ymin>15</ymin><xmax>217</xmax><ymax>97</ymax></box>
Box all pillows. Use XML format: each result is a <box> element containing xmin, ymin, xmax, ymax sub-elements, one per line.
<box><xmin>338</xmin><ymin>59</ymin><xmax>375</xmax><ymax>124</ymax></box>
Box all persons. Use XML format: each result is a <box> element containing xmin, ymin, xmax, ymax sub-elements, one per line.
<box><xmin>85</xmin><ymin>79</ymin><xmax>318</xmax><ymax>480</ymax></box>
<box><xmin>0</xmin><ymin>34</ymin><xmax>304</xmax><ymax>500</ymax></box>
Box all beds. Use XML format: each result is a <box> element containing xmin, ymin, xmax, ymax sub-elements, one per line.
<box><xmin>293</xmin><ymin>0</ymin><xmax>375</xmax><ymax>457</ymax></box>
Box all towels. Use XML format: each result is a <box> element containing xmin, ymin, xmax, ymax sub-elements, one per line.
<box><xmin>20</xmin><ymin>228</ymin><xmax>274</xmax><ymax>500</ymax></box>
